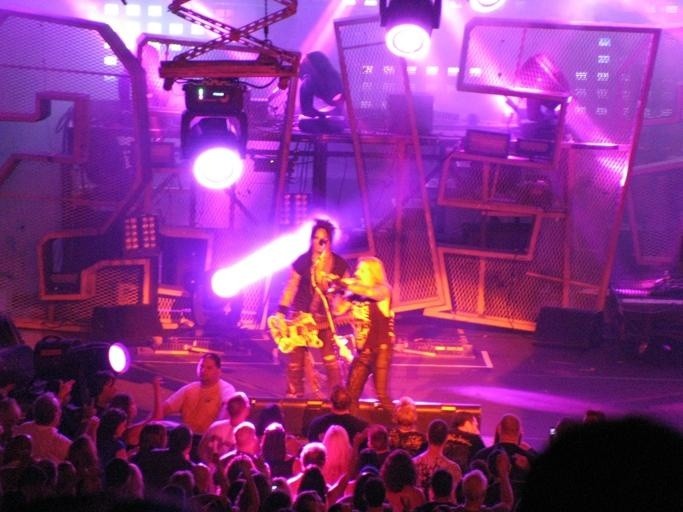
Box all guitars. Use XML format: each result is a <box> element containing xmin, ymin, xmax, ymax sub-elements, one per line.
<box><xmin>265</xmin><ymin>304</ymin><xmax>371</xmax><ymax>355</ymax></box>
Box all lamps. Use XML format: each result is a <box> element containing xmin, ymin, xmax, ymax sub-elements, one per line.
<box><xmin>179</xmin><ymin>79</ymin><xmax>248</xmax><ymax>191</ymax></box>
<box><xmin>32</xmin><ymin>335</ymin><xmax>131</xmax><ymax>379</ymax></box>
<box><xmin>378</xmin><ymin>0</ymin><xmax>441</xmax><ymax>60</ymax></box>
<box><xmin>299</xmin><ymin>51</ymin><xmax>344</xmax><ymax>134</ymax></box>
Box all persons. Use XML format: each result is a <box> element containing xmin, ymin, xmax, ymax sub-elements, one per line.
<box><xmin>1</xmin><ymin>354</ymin><xmax>683</xmax><ymax>512</ymax></box>
<box><xmin>329</xmin><ymin>256</ymin><xmax>395</xmax><ymax>412</ymax></box>
<box><xmin>276</xmin><ymin>218</ymin><xmax>344</xmax><ymax>400</ymax></box>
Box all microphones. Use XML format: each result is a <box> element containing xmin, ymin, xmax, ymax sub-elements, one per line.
<box><xmin>320</xmin><ymin>270</ymin><xmax>338</xmax><ymax>280</ymax></box>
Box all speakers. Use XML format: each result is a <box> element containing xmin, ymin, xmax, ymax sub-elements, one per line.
<box><xmin>91</xmin><ymin>303</ymin><xmax>164</xmax><ymax>343</ymax></box>
<box><xmin>533</xmin><ymin>306</ymin><xmax>604</xmax><ymax>350</ymax></box>
<box><xmin>247</xmin><ymin>395</ymin><xmax>483</xmax><ymax>444</ymax></box>
